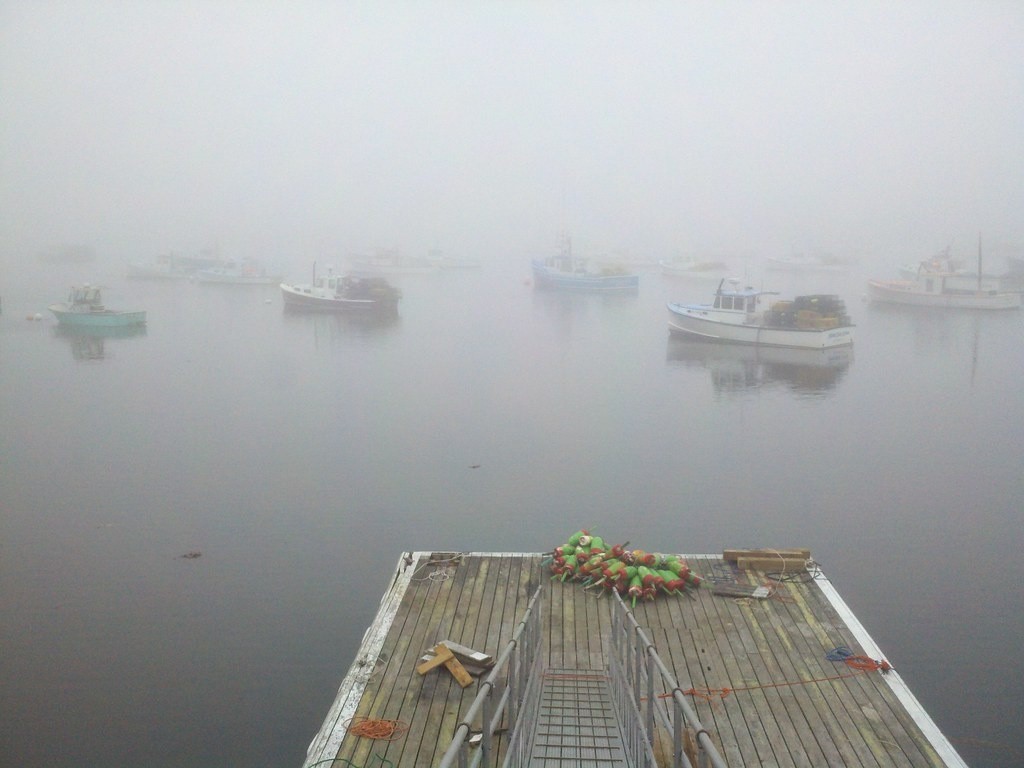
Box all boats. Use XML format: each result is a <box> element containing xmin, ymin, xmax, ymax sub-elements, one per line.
<box><xmin>198</xmin><ymin>260</ymin><xmax>275</xmax><ymax>284</ymax></box>
<box><xmin>663</xmin><ymin>273</ymin><xmax>857</xmax><ymax>351</ymax></box>
<box><xmin>279</xmin><ymin>280</ymin><xmax>399</xmax><ymax>322</ymax></box>
<box><xmin>530</xmin><ymin>252</ymin><xmax>640</xmax><ymax>296</ymax></box>
<box><xmin>46</xmin><ymin>283</ymin><xmax>146</xmax><ymax>326</ymax></box>
<box><xmin>865</xmin><ymin>227</ymin><xmax>1020</xmax><ymax>311</ymax></box>
